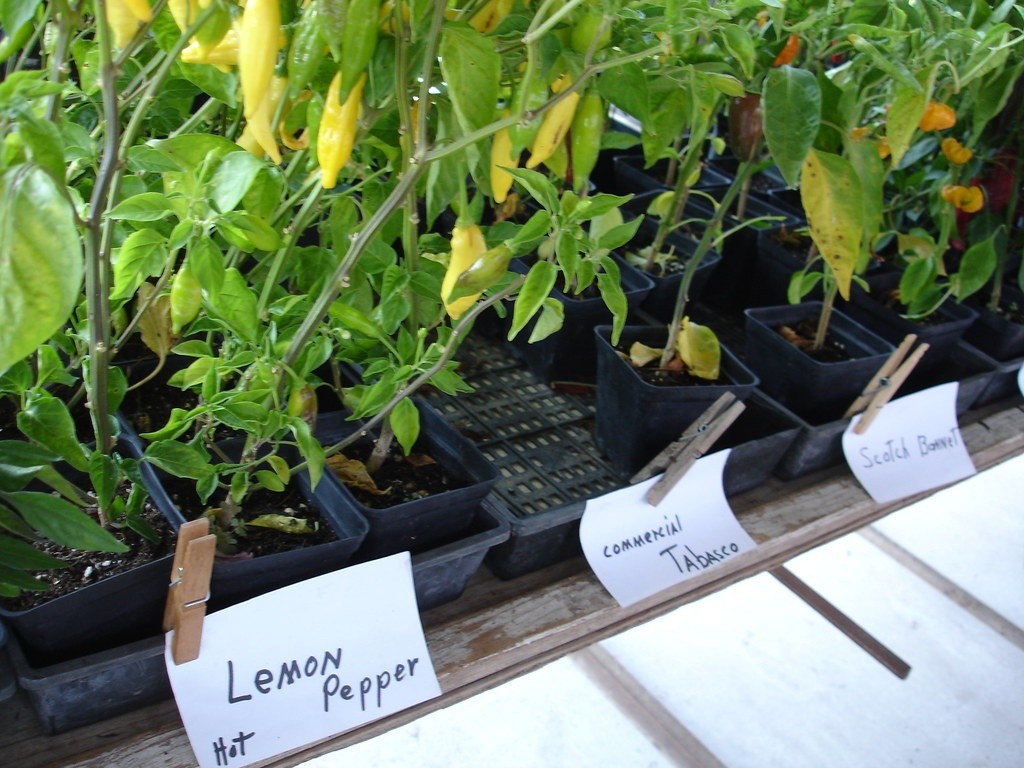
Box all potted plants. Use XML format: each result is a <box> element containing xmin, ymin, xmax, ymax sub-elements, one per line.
<box><xmin>0</xmin><ymin>0</ymin><xmax>1024</xmax><ymax>735</ymax></box>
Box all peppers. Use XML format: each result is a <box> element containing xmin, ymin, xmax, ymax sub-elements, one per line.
<box><xmin>0</xmin><ymin>0</ymin><xmax>1014</xmax><ymax>317</ymax></box>
